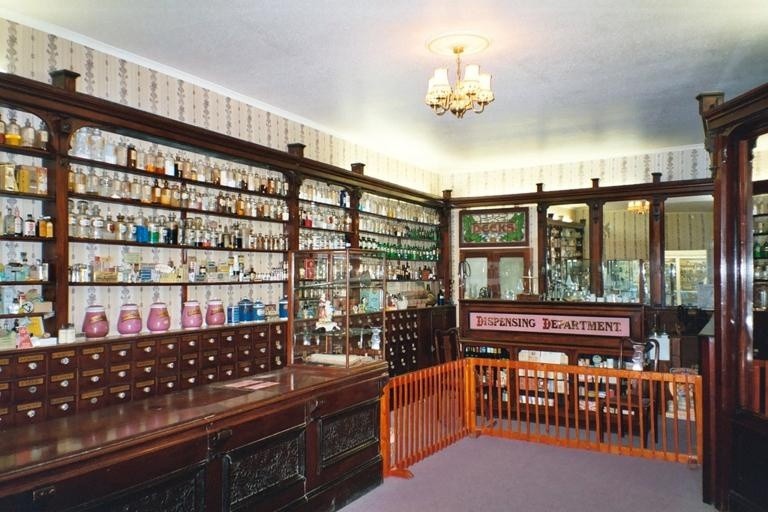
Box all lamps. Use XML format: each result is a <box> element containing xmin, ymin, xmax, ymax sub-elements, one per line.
<box><xmin>426</xmin><ymin>32</ymin><xmax>495</xmax><ymax>119</ymax></box>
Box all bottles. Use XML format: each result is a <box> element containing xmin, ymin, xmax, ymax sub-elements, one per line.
<box><xmin>426</xmin><ymin>283</ymin><xmax>436</xmax><ymax>307</ymax></box>
<box><xmin>67</xmin><ymin>323</ymin><xmax>76</xmax><ymax>344</ymax></box>
<box><xmin>751</xmin><ymin>196</ymin><xmax>768</xmax><ymax>282</ymax></box>
<box><xmin>0</xmin><ymin>113</ymin><xmax>55</xmax><ymax>281</ymax></box>
<box><xmin>357</xmin><ymin>191</ymin><xmax>441</xmax><ymax>280</ymax></box>
<box><xmin>66</xmin><ymin>126</ymin><xmax>292</xmax><ymax>281</ymax></box>
<box><xmin>57</xmin><ymin>323</ymin><xmax>66</xmax><ymax>344</ymax></box>
<box><xmin>297</xmin><ymin>182</ymin><xmax>346</xmax><ymax>316</ymax></box>
<box><xmin>13</xmin><ymin>298</ymin><xmax>19</xmax><ymax>310</ymax></box>
<box><xmin>17</xmin><ymin>291</ymin><xmax>26</xmax><ymax>306</ymax></box>
<box><xmin>438</xmin><ymin>289</ymin><xmax>444</xmax><ymax>305</ymax></box>
